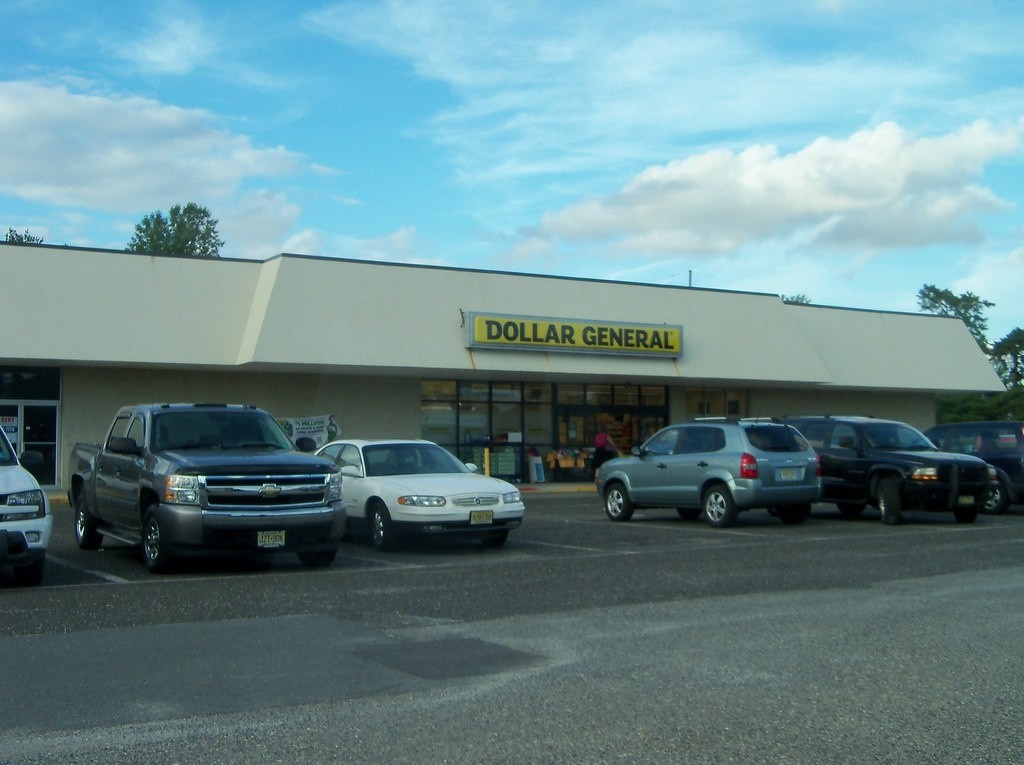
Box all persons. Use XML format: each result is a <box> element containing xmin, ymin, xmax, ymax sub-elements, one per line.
<box><xmin>326</xmin><ymin>415</ymin><xmax>337</xmax><ymax>441</ymax></box>
<box><xmin>590</xmin><ymin>423</ymin><xmax>617</xmax><ymax>471</ymax></box>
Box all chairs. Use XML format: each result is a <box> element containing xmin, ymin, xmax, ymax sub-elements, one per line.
<box><xmin>394</xmin><ymin>448</ymin><xmax>420</xmax><ymax>469</ymax></box>
<box><xmin>220</xmin><ymin>420</ymin><xmax>245</xmax><ymax>443</ymax></box>
<box><xmin>159</xmin><ymin>425</ymin><xmax>176</xmax><ymax>447</ymax></box>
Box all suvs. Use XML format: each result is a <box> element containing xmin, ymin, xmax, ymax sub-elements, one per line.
<box><xmin>767</xmin><ymin>414</ymin><xmax>1000</xmax><ymax>525</ymax></box>
<box><xmin>907</xmin><ymin>420</ymin><xmax>1024</xmax><ymax>514</ymax></box>
<box><xmin>596</xmin><ymin>414</ymin><xmax>823</xmax><ymax>528</ymax></box>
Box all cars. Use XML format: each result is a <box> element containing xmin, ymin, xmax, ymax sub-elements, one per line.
<box><xmin>313</xmin><ymin>437</ymin><xmax>525</xmax><ymax>547</ymax></box>
<box><xmin>0</xmin><ymin>425</ymin><xmax>54</xmax><ymax>568</ymax></box>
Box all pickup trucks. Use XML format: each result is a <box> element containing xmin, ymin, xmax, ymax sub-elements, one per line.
<box><xmin>66</xmin><ymin>401</ymin><xmax>349</xmax><ymax>572</ymax></box>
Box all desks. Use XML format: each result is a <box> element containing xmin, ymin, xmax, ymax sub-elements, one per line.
<box><xmin>553</xmin><ymin>456</ymin><xmax>596</xmax><ymax>483</ymax></box>
